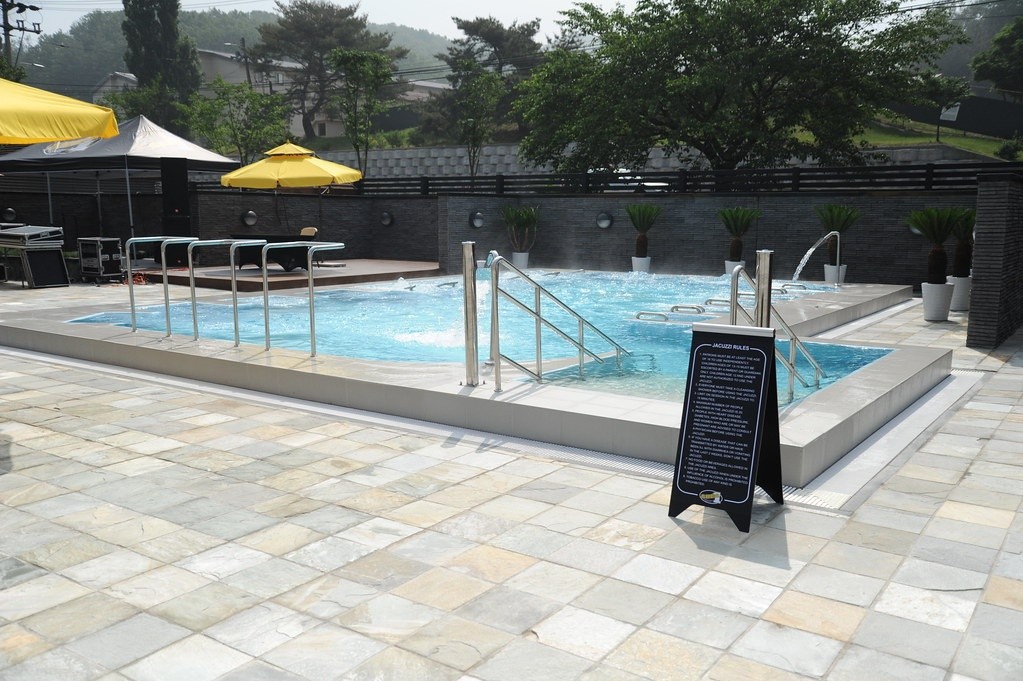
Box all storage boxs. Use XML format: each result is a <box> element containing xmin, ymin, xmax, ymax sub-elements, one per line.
<box><xmin>0</xmin><ymin>224</ymin><xmax>64</xmax><ymax>245</ymax></box>
<box><xmin>76</xmin><ymin>235</ymin><xmax>124</xmax><ymax>277</ymax></box>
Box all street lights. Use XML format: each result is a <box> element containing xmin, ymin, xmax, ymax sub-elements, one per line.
<box><xmin>225</xmin><ymin>43</ymin><xmax>273</xmax><ymax>93</ymax></box>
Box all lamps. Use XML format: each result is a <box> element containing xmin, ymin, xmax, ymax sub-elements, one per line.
<box><xmin>908</xmin><ymin>210</ymin><xmax>927</xmax><ymax>237</ymax></box>
<box><xmin>469</xmin><ymin>212</ymin><xmax>484</xmax><ymax>229</ymax></box>
<box><xmin>596</xmin><ymin>212</ymin><xmax>613</xmax><ymax>229</ymax></box>
<box><xmin>380</xmin><ymin>211</ymin><xmax>394</xmax><ymax>227</ymax></box>
<box><xmin>241</xmin><ymin>210</ymin><xmax>258</xmax><ymax>227</ymax></box>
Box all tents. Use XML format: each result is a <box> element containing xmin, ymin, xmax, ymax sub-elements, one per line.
<box><xmin>0</xmin><ymin>116</ymin><xmax>242</xmax><ymax>265</ymax></box>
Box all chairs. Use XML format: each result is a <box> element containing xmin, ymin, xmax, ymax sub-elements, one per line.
<box><xmin>301</xmin><ymin>227</ymin><xmax>320</xmax><ymax>270</ymax></box>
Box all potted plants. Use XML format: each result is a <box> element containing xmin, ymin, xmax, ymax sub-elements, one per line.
<box><xmin>496</xmin><ymin>203</ymin><xmax>542</xmax><ymax>269</ymax></box>
<box><xmin>714</xmin><ymin>206</ymin><xmax>762</xmax><ymax>276</ymax></box>
<box><xmin>624</xmin><ymin>203</ymin><xmax>663</xmax><ymax>272</ymax></box>
<box><xmin>905</xmin><ymin>204</ymin><xmax>975</xmax><ymax>322</ymax></box>
<box><xmin>815</xmin><ymin>202</ymin><xmax>867</xmax><ymax>283</ymax></box>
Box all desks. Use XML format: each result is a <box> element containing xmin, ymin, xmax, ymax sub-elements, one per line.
<box><xmin>231</xmin><ymin>234</ymin><xmax>314</xmax><ymax>272</ymax></box>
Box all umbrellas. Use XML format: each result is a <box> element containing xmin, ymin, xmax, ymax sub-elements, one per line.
<box><xmin>0</xmin><ymin>78</ymin><xmax>119</xmax><ymax>139</ymax></box>
<box><xmin>221</xmin><ymin>142</ymin><xmax>362</xmax><ymax>263</ymax></box>
